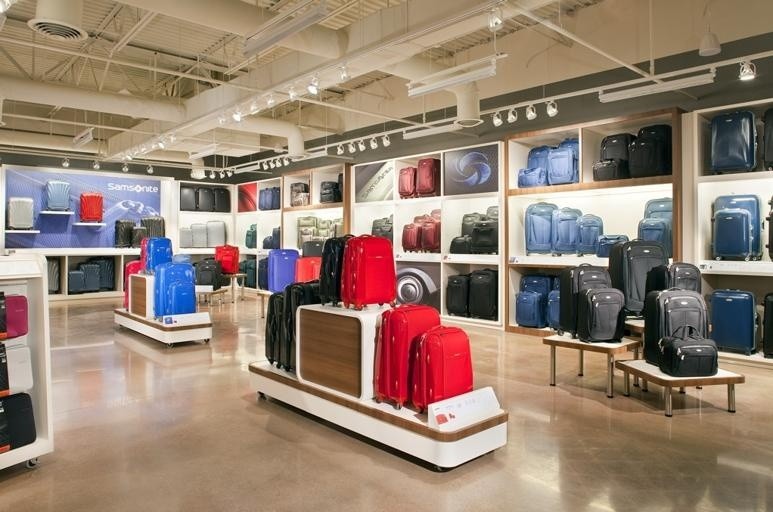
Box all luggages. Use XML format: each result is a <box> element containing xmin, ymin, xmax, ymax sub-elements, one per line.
<box><xmin>180</xmin><ymin>186</ymin><xmax>230</xmax><ymax>211</ymax></box>
<box><xmin>259</xmin><ymin>173</ymin><xmax>343</xmax><ymax>211</ymax></box>
<box><xmin>48</xmin><ymin>259</ymin><xmax>60</xmax><ymax>294</ymax></box>
<box><xmin>517</xmin><ymin>106</ymin><xmax>773</xmax><ymax>377</ymax></box>
<box><xmin>242</xmin><ymin>222</ymin><xmax>281</xmax><ymax>293</ymax></box>
<box><xmin>265</xmin><ymin>232</ymin><xmax>474</xmax><ymax>412</ymax></box>
<box><xmin>68</xmin><ymin>215</ymin><xmax>239</xmax><ymax>323</ymax></box>
<box><xmin>372</xmin><ymin>217</ymin><xmax>393</xmax><ymax>242</ymax></box>
<box><xmin>8</xmin><ymin>180</ymin><xmax>104</xmax><ymax>229</ymax></box>
<box><xmin>297</xmin><ymin>218</ymin><xmax>343</xmax><ymax>251</ymax></box>
<box><xmin>449</xmin><ymin>206</ymin><xmax>499</xmax><ymax>254</ymax></box>
<box><xmin>401</xmin><ymin>211</ymin><xmax>441</xmax><ymax>253</ymax></box>
<box><xmin>446</xmin><ymin>267</ymin><xmax>497</xmax><ymax>322</ymax></box>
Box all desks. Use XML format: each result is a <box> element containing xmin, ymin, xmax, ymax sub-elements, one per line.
<box><xmin>542</xmin><ymin>332</ymin><xmax>639</xmax><ymax>397</ymax></box>
<box><xmin>615</xmin><ymin>358</ymin><xmax>744</xmax><ymax>417</ymax></box>
<box><xmin>620</xmin><ymin>314</ymin><xmax>712</xmax><ymax>392</ymax></box>
<box><xmin>193</xmin><ymin>272</ymin><xmax>271</xmax><ymax>318</ymax></box>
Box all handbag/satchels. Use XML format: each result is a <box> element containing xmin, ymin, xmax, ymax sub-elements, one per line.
<box><xmin>0</xmin><ymin>295</ymin><xmax>37</xmax><ymax>453</ymax></box>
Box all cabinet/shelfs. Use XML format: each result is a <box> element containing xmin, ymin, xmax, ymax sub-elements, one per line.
<box><xmin>176</xmin><ymin>177</ymin><xmax>233</xmax><ymax>288</ymax></box>
<box><xmin>279</xmin><ymin>162</ymin><xmax>349</xmax><ymax>258</ymax></box>
<box><xmin>504</xmin><ymin>106</ymin><xmax>686</xmax><ymax>339</ymax></box>
<box><xmin>682</xmin><ymin>98</ymin><xmax>773</xmax><ymax>370</ymax></box>
<box><xmin>112</xmin><ymin>274</ymin><xmax>212</xmax><ymax>348</ymax></box>
<box><xmin>0</xmin><ymin>254</ymin><xmax>54</xmax><ymax>473</ymax></box>
<box><xmin>248</xmin><ymin>301</ymin><xmax>507</xmax><ymax>472</ymax></box>
<box><xmin>352</xmin><ymin>140</ymin><xmax>502</xmax><ymax>330</ymax></box>
<box><xmin>234</xmin><ymin>178</ymin><xmax>279</xmax><ymax>295</ymax></box>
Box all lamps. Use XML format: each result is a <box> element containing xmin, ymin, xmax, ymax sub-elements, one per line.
<box><xmin>233</xmin><ymin>109</ymin><xmax>241</xmax><ymax>121</ymax></box>
<box><xmin>122</xmin><ymin>163</ymin><xmax>129</xmax><ymax>173</ymax></box>
<box><xmin>72</xmin><ymin>127</ymin><xmax>95</xmax><ymax>147</ymax></box>
<box><xmin>288</xmin><ymin>87</ymin><xmax>299</xmax><ymax>102</ymax></box>
<box><xmin>62</xmin><ymin>157</ymin><xmax>70</xmax><ymax>168</ymax></box>
<box><xmin>93</xmin><ymin>160</ymin><xmax>101</xmax><ymax>170</ymax></box>
<box><xmin>698</xmin><ymin>4</ymin><xmax>721</xmax><ymax>56</ymax></box>
<box><xmin>336</xmin><ymin>136</ymin><xmax>392</xmax><ymax>156</ymax></box>
<box><xmin>250</xmin><ymin>102</ymin><xmax>261</xmax><ymax>115</ymax></box>
<box><xmin>338</xmin><ymin>65</ymin><xmax>350</xmax><ymax>81</ymax></box>
<box><xmin>126</xmin><ymin>134</ymin><xmax>177</xmax><ymax>162</ymax></box>
<box><xmin>146</xmin><ymin>165</ymin><xmax>154</xmax><ymax>175</ymax></box>
<box><xmin>599</xmin><ymin>73</ymin><xmax>714</xmax><ymax>103</ymax></box>
<box><xmin>306</xmin><ymin>76</ymin><xmax>321</xmax><ymax>95</ymax></box>
<box><xmin>188</xmin><ymin>144</ymin><xmax>219</xmax><ymax>160</ymax></box>
<box><xmin>267</xmin><ymin>95</ymin><xmax>276</xmax><ymax>108</ymax></box>
<box><xmin>737</xmin><ymin>59</ymin><xmax>757</xmax><ymax>82</ymax></box>
<box><xmin>219</xmin><ymin>114</ymin><xmax>227</xmax><ymax>124</ymax></box>
<box><xmin>208</xmin><ymin>171</ymin><xmax>233</xmax><ymax>180</ymax></box>
<box><xmin>404</xmin><ymin>50</ymin><xmax>509</xmax><ymax>99</ymax></box>
<box><xmin>262</xmin><ymin>158</ymin><xmax>290</xmax><ymax>171</ymax></box>
<box><xmin>492</xmin><ymin>100</ymin><xmax>558</xmax><ymax>128</ymax></box>
